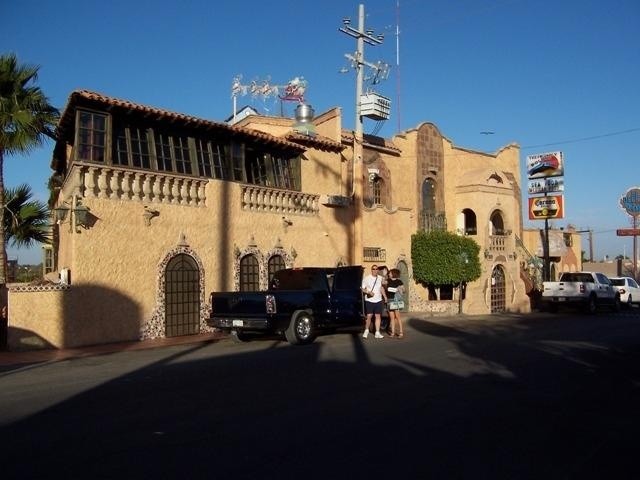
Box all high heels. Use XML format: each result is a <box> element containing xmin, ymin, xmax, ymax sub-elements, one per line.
<box><xmin>389</xmin><ymin>332</ymin><xmax>404</xmax><ymax>339</ymax></box>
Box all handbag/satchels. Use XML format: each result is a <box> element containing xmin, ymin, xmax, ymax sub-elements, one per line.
<box><xmin>367</xmin><ymin>294</ymin><xmax>373</xmax><ymax>298</ymax></box>
<box><xmin>390</xmin><ymin>301</ymin><xmax>404</xmax><ymax>310</ymax></box>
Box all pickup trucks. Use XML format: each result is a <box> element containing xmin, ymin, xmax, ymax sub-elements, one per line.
<box><xmin>542</xmin><ymin>271</ymin><xmax>640</xmax><ymax>315</ymax></box>
<box><xmin>205</xmin><ymin>266</ymin><xmax>390</xmax><ymax>346</ymax></box>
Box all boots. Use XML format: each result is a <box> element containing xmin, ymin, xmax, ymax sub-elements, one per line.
<box><xmin>362</xmin><ymin>329</ymin><xmax>369</xmax><ymax>339</ymax></box>
<box><xmin>375</xmin><ymin>332</ymin><xmax>384</xmax><ymax>339</ymax></box>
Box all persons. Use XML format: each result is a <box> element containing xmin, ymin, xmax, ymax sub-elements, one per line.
<box><xmin>381</xmin><ymin>267</ymin><xmax>408</xmax><ymax>339</ymax></box>
<box><xmin>361</xmin><ymin>264</ymin><xmax>389</xmax><ymax>339</ymax></box>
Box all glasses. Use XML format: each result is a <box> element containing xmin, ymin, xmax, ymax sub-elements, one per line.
<box><xmin>371</xmin><ymin>268</ymin><xmax>379</xmax><ymax>271</ymax></box>
